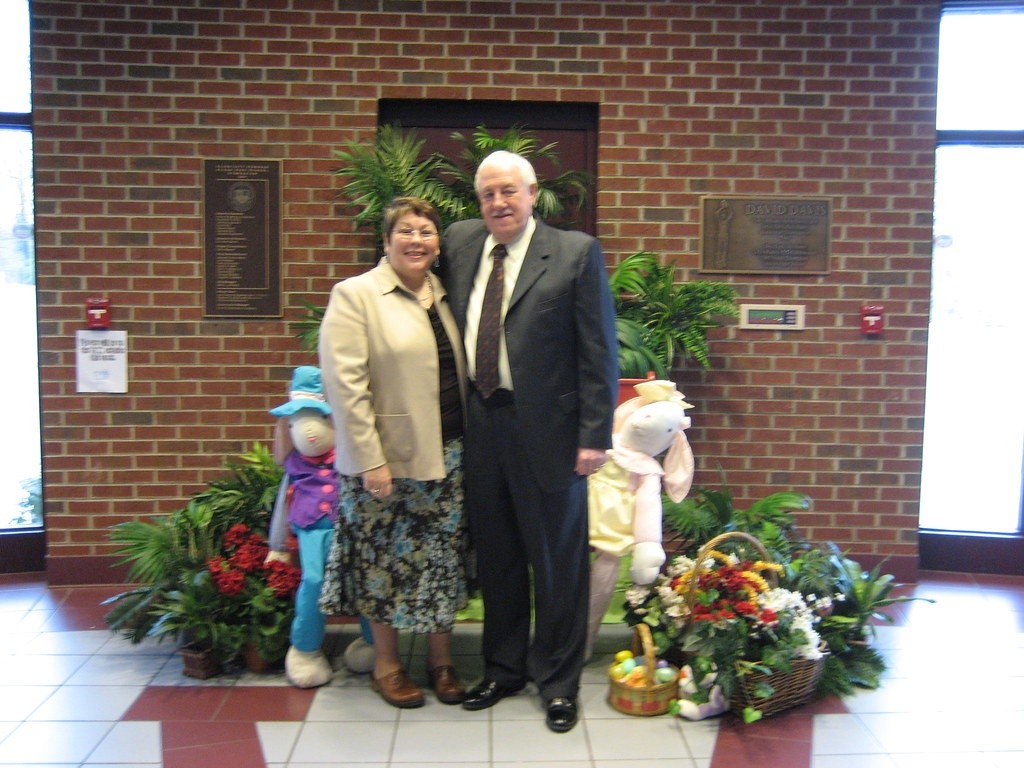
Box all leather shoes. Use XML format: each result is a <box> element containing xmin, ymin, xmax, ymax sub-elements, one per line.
<box><xmin>463</xmin><ymin>676</ymin><xmax>525</xmax><ymax>712</ymax></box>
<box><xmin>547</xmin><ymin>696</ymin><xmax>578</xmax><ymax>732</ymax></box>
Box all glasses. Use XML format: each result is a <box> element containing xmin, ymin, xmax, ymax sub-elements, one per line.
<box><xmin>387</xmin><ymin>226</ymin><xmax>441</xmax><ymax>241</ymax></box>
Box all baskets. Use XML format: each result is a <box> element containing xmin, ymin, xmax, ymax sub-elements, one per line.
<box><xmin>691</xmin><ymin>532</ymin><xmax>830</xmax><ymax>718</ymax></box>
<box><xmin>608</xmin><ymin>623</ymin><xmax>681</xmax><ymax>717</ymax></box>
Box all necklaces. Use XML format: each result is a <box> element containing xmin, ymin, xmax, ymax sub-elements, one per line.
<box><xmin>417</xmin><ymin>273</ymin><xmax>433</xmax><ymax>301</ymax></box>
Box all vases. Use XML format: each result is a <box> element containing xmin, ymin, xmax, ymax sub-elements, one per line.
<box><xmin>218</xmin><ymin>644</ymin><xmax>247</xmax><ymax>676</ymax></box>
<box><xmin>250</xmin><ymin>640</ymin><xmax>272</xmax><ymax>673</ymax></box>
<box><xmin>179</xmin><ymin>640</ymin><xmax>216</xmax><ymax>679</ymax></box>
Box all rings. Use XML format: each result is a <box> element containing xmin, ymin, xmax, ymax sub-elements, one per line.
<box><xmin>373</xmin><ymin>489</ymin><xmax>380</xmax><ymax>494</ymax></box>
<box><xmin>600</xmin><ymin>464</ymin><xmax>606</xmax><ymax>467</ymax></box>
<box><xmin>593</xmin><ymin>467</ymin><xmax>599</xmax><ymax>472</ymax></box>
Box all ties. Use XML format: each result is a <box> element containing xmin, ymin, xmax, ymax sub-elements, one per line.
<box><xmin>476</xmin><ymin>244</ymin><xmax>508</xmax><ymax>399</ymax></box>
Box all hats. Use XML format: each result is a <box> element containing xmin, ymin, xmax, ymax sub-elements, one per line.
<box><xmin>270</xmin><ymin>366</ymin><xmax>333</xmax><ymax>417</ymax></box>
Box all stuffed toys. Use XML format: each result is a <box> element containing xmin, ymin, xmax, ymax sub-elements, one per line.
<box><xmin>582</xmin><ymin>379</ymin><xmax>695</xmax><ymax>665</ymax></box>
<box><xmin>264</xmin><ymin>365</ymin><xmax>376</xmax><ymax>688</ymax></box>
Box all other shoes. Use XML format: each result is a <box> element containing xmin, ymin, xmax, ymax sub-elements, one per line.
<box><xmin>429</xmin><ymin>665</ymin><xmax>465</xmax><ymax>704</ymax></box>
<box><xmin>373</xmin><ymin>668</ymin><xmax>424</xmax><ymax>708</ymax></box>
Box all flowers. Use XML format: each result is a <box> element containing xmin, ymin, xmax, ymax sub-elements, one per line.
<box><xmin>100</xmin><ymin>438</ymin><xmax>300</xmax><ymax>657</ymax></box>
<box><xmin>621</xmin><ymin>547</ymin><xmax>846</xmax><ymax>721</ymax></box>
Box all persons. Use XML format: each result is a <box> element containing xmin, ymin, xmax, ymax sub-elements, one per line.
<box><xmin>436</xmin><ymin>151</ymin><xmax>618</xmax><ymax>733</ymax></box>
<box><xmin>317</xmin><ymin>196</ymin><xmax>471</xmax><ymax>709</ymax></box>
